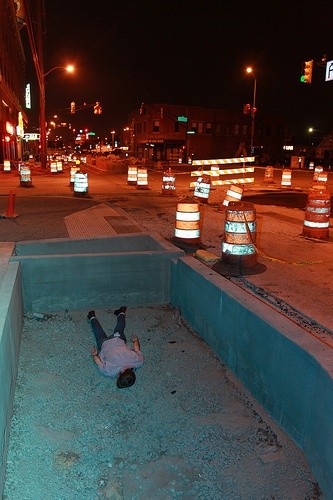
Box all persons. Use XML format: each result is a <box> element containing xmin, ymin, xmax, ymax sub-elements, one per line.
<box><xmin>87</xmin><ymin>306</ymin><xmax>144</xmax><ymax>389</ymax></box>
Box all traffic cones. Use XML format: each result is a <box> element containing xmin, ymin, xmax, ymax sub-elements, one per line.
<box><xmin>0</xmin><ymin>191</ymin><xmax>19</xmax><ymax>219</ymax></box>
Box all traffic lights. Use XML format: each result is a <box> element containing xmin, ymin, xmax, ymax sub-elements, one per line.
<box><xmin>301</xmin><ymin>59</ymin><xmax>313</xmax><ymax>84</ymax></box>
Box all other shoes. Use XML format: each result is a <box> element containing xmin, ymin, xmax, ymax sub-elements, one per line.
<box><xmin>88</xmin><ymin>311</ymin><xmax>95</xmax><ymax>320</ymax></box>
<box><xmin>114</xmin><ymin>306</ymin><xmax>126</xmax><ymax>315</ymax></box>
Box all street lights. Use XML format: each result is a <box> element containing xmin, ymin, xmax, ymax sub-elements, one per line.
<box><xmin>247</xmin><ymin>67</ymin><xmax>256</xmax><ymax>156</ymax></box>
<box><xmin>39</xmin><ymin>64</ymin><xmax>75</xmax><ymax>172</ymax></box>
